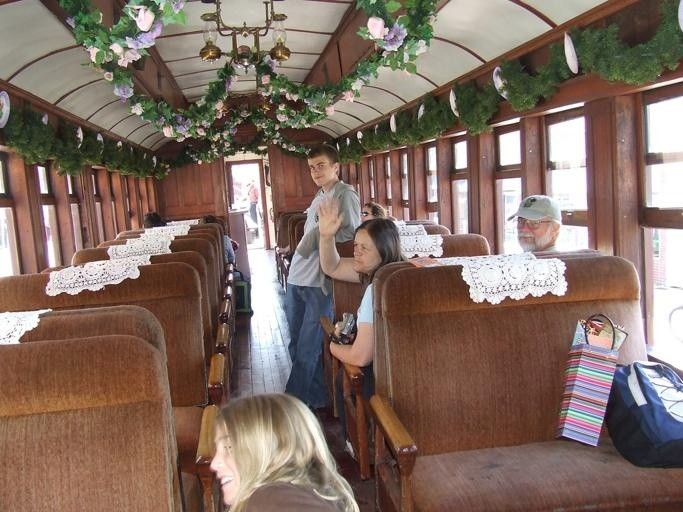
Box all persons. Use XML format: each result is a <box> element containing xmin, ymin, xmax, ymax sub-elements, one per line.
<box><xmin>144</xmin><ymin>212</ymin><xmax>167</xmax><ymax>228</ymax></box>
<box><xmin>199</xmin><ymin>215</ymin><xmax>236</xmax><ymax>264</ymax></box>
<box><xmin>248</xmin><ymin>180</ymin><xmax>258</xmax><ymax>223</ymax></box>
<box><xmin>507</xmin><ymin>194</ymin><xmax>562</xmax><ymax>252</ymax></box>
<box><xmin>284</xmin><ymin>143</ymin><xmax>362</xmax><ymax>409</ymax></box>
<box><xmin>208</xmin><ymin>393</ymin><xmax>360</xmax><ymax>512</ymax></box>
<box><xmin>316</xmin><ymin>197</ymin><xmax>408</xmax><ymax>441</ymax></box>
<box><xmin>360</xmin><ymin>202</ymin><xmax>386</xmax><ymax>223</ymax></box>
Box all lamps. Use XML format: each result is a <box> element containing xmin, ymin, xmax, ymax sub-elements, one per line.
<box><xmin>198</xmin><ymin>0</ymin><xmax>291</xmax><ymax>74</ymax></box>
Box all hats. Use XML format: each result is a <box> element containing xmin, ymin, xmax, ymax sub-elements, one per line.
<box><xmin>508</xmin><ymin>195</ymin><xmax>562</xmax><ymax>221</ymax></box>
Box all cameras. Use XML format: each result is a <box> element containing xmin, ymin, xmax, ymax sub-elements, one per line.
<box><xmin>334</xmin><ymin>312</ymin><xmax>356</xmax><ymax>337</ymax></box>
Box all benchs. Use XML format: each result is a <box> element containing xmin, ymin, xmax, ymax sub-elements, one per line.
<box><xmin>0</xmin><ymin>262</ymin><xmax>224</xmax><ymax>512</ymax></box>
<box><xmin>340</xmin><ymin>249</ymin><xmax>608</xmax><ymax>480</ymax></box>
<box><xmin>319</xmin><ymin>234</ymin><xmax>490</xmax><ymax>418</ymax></box>
<box><xmin>275</xmin><ymin>210</ymin><xmax>452</xmax><ymax>294</ymax></box>
<box><xmin>0</xmin><ymin>306</ymin><xmax>185</xmax><ymax>512</ymax></box>
<box><xmin>40</xmin><ymin>216</ymin><xmax>234</xmax><ymax>406</ymax></box>
<box><xmin>0</xmin><ymin>335</ymin><xmax>175</xmax><ymax>512</ymax></box>
<box><xmin>368</xmin><ymin>255</ymin><xmax>683</xmax><ymax>512</ymax></box>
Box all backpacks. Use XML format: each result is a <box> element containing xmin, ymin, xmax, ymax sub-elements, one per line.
<box><xmin>605</xmin><ymin>361</ymin><xmax>683</xmax><ymax>467</ymax></box>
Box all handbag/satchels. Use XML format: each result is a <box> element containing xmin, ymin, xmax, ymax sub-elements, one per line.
<box><xmin>341</xmin><ymin>312</ymin><xmax>357</xmax><ymax>345</ymax></box>
<box><xmin>555</xmin><ymin>343</ymin><xmax>620</xmax><ymax>447</ymax></box>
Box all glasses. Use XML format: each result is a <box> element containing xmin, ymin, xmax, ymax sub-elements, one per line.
<box><xmin>513</xmin><ymin>218</ymin><xmax>551</xmax><ymax>230</ymax></box>
<box><xmin>360</xmin><ymin>212</ymin><xmax>373</xmax><ymax>217</ymax></box>
